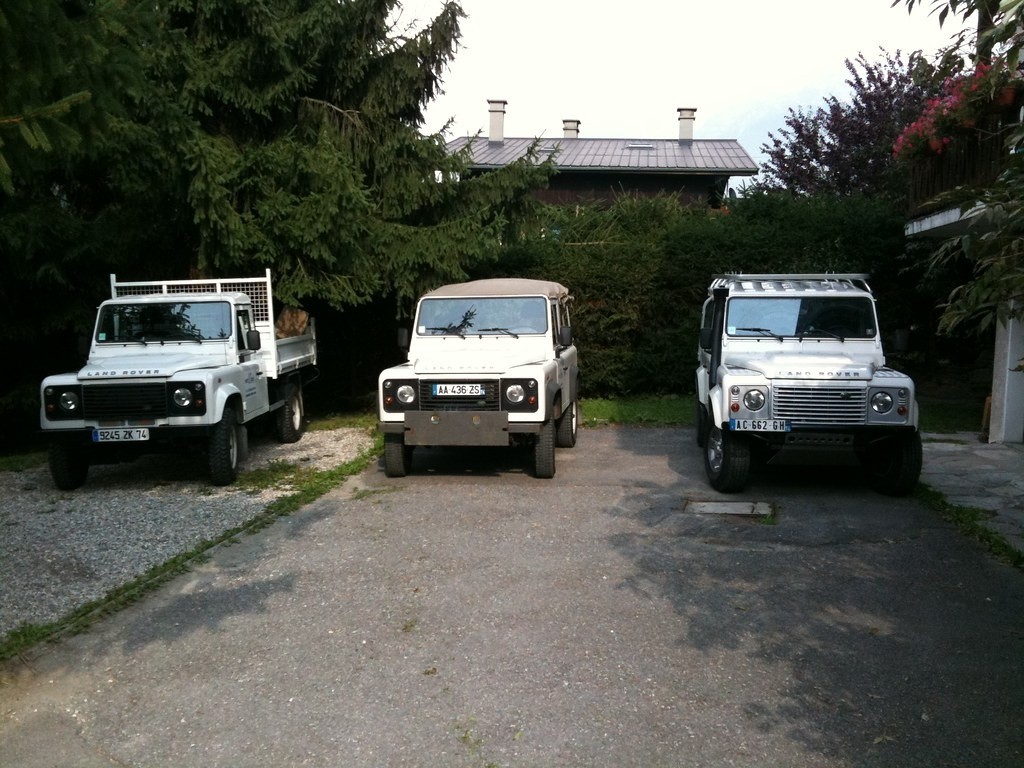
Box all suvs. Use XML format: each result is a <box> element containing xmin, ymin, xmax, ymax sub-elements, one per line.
<box><xmin>376</xmin><ymin>277</ymin><xmax>579</xmax><ymax>478</ymax></box>
<box><xmin>696</xmin><ymin>270</ymin><xmax>923</xmax><ymax>495</ymax></box>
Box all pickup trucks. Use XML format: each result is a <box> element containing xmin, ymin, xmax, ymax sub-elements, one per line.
<box><xmin>39</xmin><ymin>267</ymin><xmax>317</xmax><ymax>491</ymax></box>
